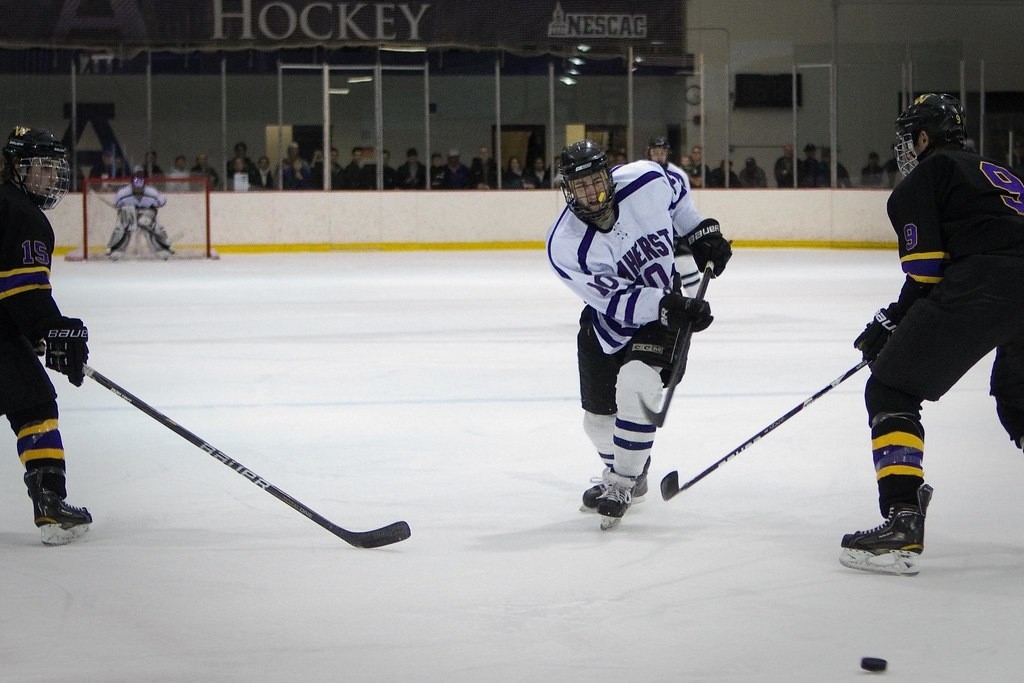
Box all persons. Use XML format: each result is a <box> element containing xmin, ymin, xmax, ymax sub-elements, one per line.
<box><xmin>839</xmin><ymin>94</ymin><xmax>1024</xmax><ymax>576</ymax></box>
<box><xmin>605</xmin><ymin>148</ymin><xmax>626</xmax><ymax>165</ymax></box>
<box><xmin>545</xmin><ymin>138</ymin><xmax>733</xmax><ymax>530</ymax></box>
<box><xmin>88</xmin><ymin>150</ymin><xmax>218</xmax><ymax>191</ymax></box>
<box><xmin>1014</xmin><ymin>142</ymin><xmax>1024</xmax><ymax>176</ymax></box>
<box><xmin>774</xmin><ymin>144</ymin><xmax>852</xmax><ymax>188</ymax></box>
<box><xmin>860</xmin><ymin>152</ymin><xmax>903</xmax><ymax>186</ymax></box>
<box><xmin>106</xmin><ymin>172</ymin><xmax>175</xmax><ymax>261</ymax></box>
<box><xmin>0</xmin><ymin>127</ymin><xmax>93</xmax><ymax>546</ymax></box>
<box><xmin>647</xmin><ymin>136</ymin><xmax>701</xmax><ymax>296</ymax></box>
<box><xmin>227</xmin><ymin>142</ymin><xmax>565</xmax><ymax>190</ymax></box>
<box><xmin>681</xmin><ymin>147</ymin><xmax>767</xmax><ymax>188</ymax></box>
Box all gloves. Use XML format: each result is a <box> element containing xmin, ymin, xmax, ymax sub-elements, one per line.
<box><xmin>854</xmin><ymin>303</ymin><xmax>907</xmax><ymax>361</ymax></box>
<box><xmin>46</xmin><ymin>316</ymin><xmax>89</xmax><ymax>388</ymax></box>
<box><xmin>680</xmin><ymin>218</ymin><xmax>732</xmax><ymax>279</ymax></box>
<box><xmin>659</xmin><ymin>287</ymin><xmax>714</xmax><ymax>332</ymax></box>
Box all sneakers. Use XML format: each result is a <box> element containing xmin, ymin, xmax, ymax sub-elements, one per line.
<box><xmin>579</xmin><ymin>455</ymin><xmax>651</xmax><ymax>512</ymax></box>
<box><xmin>839</xmin><ymin>484</ymin><xmax>934</xmax><ymax>575</ymax></box>
<box><xmin>596</xmin><ymin>474</ymin><xmax>643</xmax><ymax>530</ymax></box>
<box><xmin>29</xmin><ymin>486</ymin><xmax>92</xmax><ymax>546</ymax></box>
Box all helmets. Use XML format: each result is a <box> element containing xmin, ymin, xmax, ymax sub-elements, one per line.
<box><xmin>647</xmin><ymin>135</ymin><xmax>671</xmax><ymax>148</ymax></box>
<box><xmin>0</xmin><ymin>125</ymin><xmax>71</xmax><ymax>210</ymax></box>
<box><xmin>558</xmin><ymin>140</ymin><xmax>615</xmax><ymax>219</ymax></box>
<box><xmin>894</xmin><ymin>93</ymin><xmax>967</xmax><ymax>177</ymax></box>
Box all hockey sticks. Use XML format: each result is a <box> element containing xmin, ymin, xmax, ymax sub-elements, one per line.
<box><xmin>636</xmin><ymin>262</ymin><xmax>716</xmax><ymax>429</ymax></box>
<box><xmin>89</xmin><ymin>187</ymin><xmax>185</xmax><ymax>245</ymax></box>
<box><xmin>659</xmin><ymin>357</ymin><xmax>869</xmax><ymax>502</ymax></box>
<box><xmin>81</xmin><ymin>364</ymin><xmax>412</xmax><ymax>550</ymax></box>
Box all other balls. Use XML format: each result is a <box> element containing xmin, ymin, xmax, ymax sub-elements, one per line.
<box><xmin>860</xmin><ymin>657</ymin><xmax>887</xmax><ymax>672</ymax></box>
